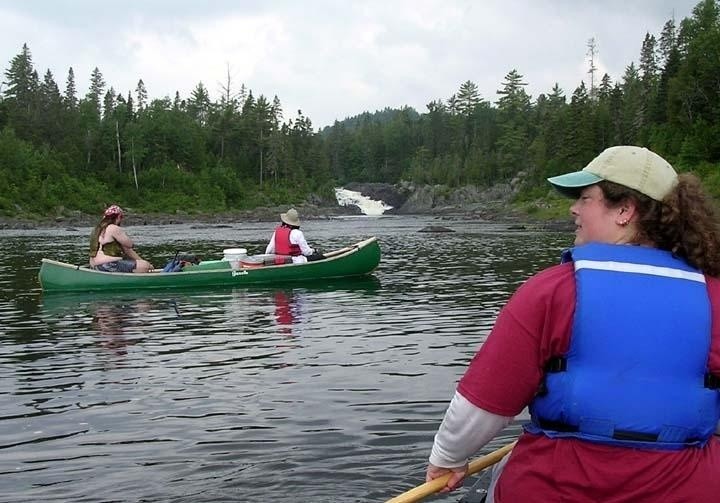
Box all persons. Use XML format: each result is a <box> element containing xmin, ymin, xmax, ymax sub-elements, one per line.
<box><xmin>264</xmin><ymin>207</ymin><xmax>318</xmax><ymax>256</ymax></box>
<box><xmin>88</xmin><ymin>204</ymin><xmax>155</xmax><ymax>273</ymax></box>
<box><xmin>421</xmin><ymin>142</ymin><xmax>720</xmax><ymax>503</ymax></box>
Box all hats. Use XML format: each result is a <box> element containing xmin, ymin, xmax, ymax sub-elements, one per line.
<box><xmin>105</xmin><ymin>204</ymin><xmax>124</xmax><ymax>217</ymax></box>
<box><xmin>546</xmin><ymin>144</ymin><xmax>680</xmax><ymax>203</ymax></box>
<box><xmin>280</xmin><ymin>209</ymin><xmax>300</xmax><ymax>228</ymax></box>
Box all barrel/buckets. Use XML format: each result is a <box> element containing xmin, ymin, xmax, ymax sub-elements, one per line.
<box><xmin>224</xmin><ymin>248</ymin><xmax>247</xmax><ymax>268</ymax></box>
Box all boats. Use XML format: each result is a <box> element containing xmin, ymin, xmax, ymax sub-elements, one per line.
<box><xmin>38</xmin><ymin>236</ymin><xmax>381</xmax><ymax>296</ymax></box>
